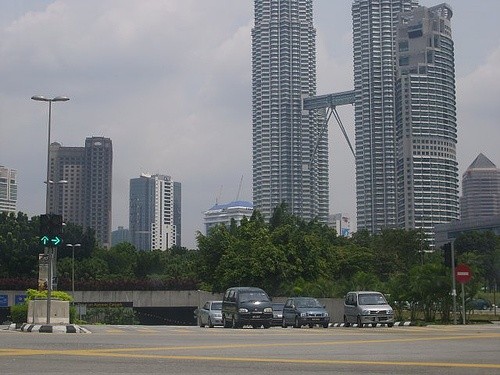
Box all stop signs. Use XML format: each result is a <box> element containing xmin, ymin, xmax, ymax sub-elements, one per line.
<box><xmin>454</xmin><ymin>263</ymin><xmax>472</xmax><ymax>282</ymax></box>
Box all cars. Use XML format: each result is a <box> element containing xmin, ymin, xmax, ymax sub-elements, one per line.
<box><xmin>344</xmin><ymin>289</ymin><xmax>394</xmax><ymax>328</ymax></box>
<box><xmin>388</xmin><ymin>295</ymin><xmax>490</xmax><ymax>310</ymax></box>
<box><xmin>270</xmin><ymin>303</ymin><xmax>286</xmax><ymax>326</ymax></box>
<box><xmin>282</xmin><ymin>296</ymin><xmax>331</xmax><ymax>329</ymax></box>
<box><xmin>197</xmin><ymin>300</ymin><xmax>224</xmax><ymax>330</ymax></box>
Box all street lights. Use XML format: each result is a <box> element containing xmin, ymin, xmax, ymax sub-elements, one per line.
<box><xmin>66</xmin><ymin>243</ymin><xmax>83</xmax><ymax>322</ymax></box>
<box><xmin>42</xmin><ymin>179</ymin><xmax>67</xmax><ymax>289</ymax></box>
<box><xmin>31</xmin><ymin>94</ymin><xmax>74</xmax><ymax>324</ymax></box>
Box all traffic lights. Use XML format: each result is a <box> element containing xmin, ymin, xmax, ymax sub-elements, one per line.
<box><xmin>441</xmin><ymin>242</ymin><xmax>452</xmax><ymax>268</ymax></box>
<box><xmin>51</xmin><ymin>214</ymin><xmax>62</xmax><ymax>246</ymax></box>
<box><xmin>40</xmin><ymin>214</ymin><xmax>51</xmax><ymax>246</ymax></box>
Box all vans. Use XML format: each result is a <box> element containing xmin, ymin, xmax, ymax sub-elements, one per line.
<box><xmin>221</xmin><ymin>286</ymin><xmax>272</xmax><ymax>329</ymax></box>
